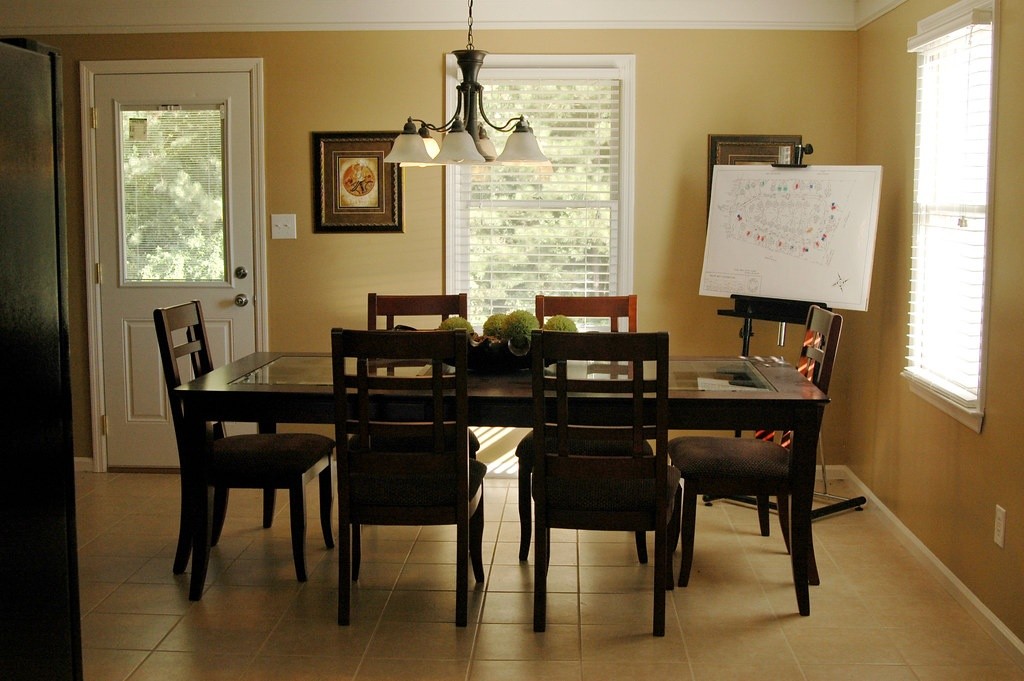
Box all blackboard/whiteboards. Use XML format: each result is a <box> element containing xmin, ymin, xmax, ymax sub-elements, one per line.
<box><xmin>699</xmin><ymin>166</ymin><xmax>882</xmax><ymax>312</ymax></box>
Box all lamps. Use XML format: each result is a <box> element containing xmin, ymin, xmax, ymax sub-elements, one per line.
<box><xmin>383</xmin><ymin>0</ymin><xmax>549</xmax><ymax>166</ymax></box>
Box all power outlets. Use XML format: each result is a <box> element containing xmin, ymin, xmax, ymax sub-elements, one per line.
<box><xmin>991</xmin><ymin>504</ymin><xmax>1007</xmax><ymax>549</ymax></box>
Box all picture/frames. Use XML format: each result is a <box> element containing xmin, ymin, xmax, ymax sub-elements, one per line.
<box><xmin>707</xmin><ymin>134</ymin><xmax>802</xmax><ymax>227</ymax></box>
<box><xmin>309</xmin><ymin>129</ymin><xmax>404</xmax><ymax>235</ymax></box>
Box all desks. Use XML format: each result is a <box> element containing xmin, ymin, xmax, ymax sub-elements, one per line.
<box><xmin>170</xmin><ymin>350</ymin><xmax>830</xmax><ymax>616</ymax></box>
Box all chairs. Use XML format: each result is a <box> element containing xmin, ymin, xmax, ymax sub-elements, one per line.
<box><xmin>152</xmin><ymin>298</ymin><xmax>335</xmax><ymax>607</ymax></box>
<box><xmin>329</xmin><ymin>328</ymin><xmax>487</xmax><ymax>628</ymax></box>
<box><xmin>533</xmin><ymin>293</ymin><xmax>640</xmax><ymax>361</ymax></box>
<box><xmin>366</xmin><ymin>291</ymin><xmax>468</xmax><ymax>331</ymax></box>
<box><xmin>669</xmin><ymin>306</ymin><xmax>843</xmax><ymax>616</ymax></box>
<box><xmin>525</xmin><ymin>331</ymin><xmax>681</xmax><ymax>638</ymax></box>
